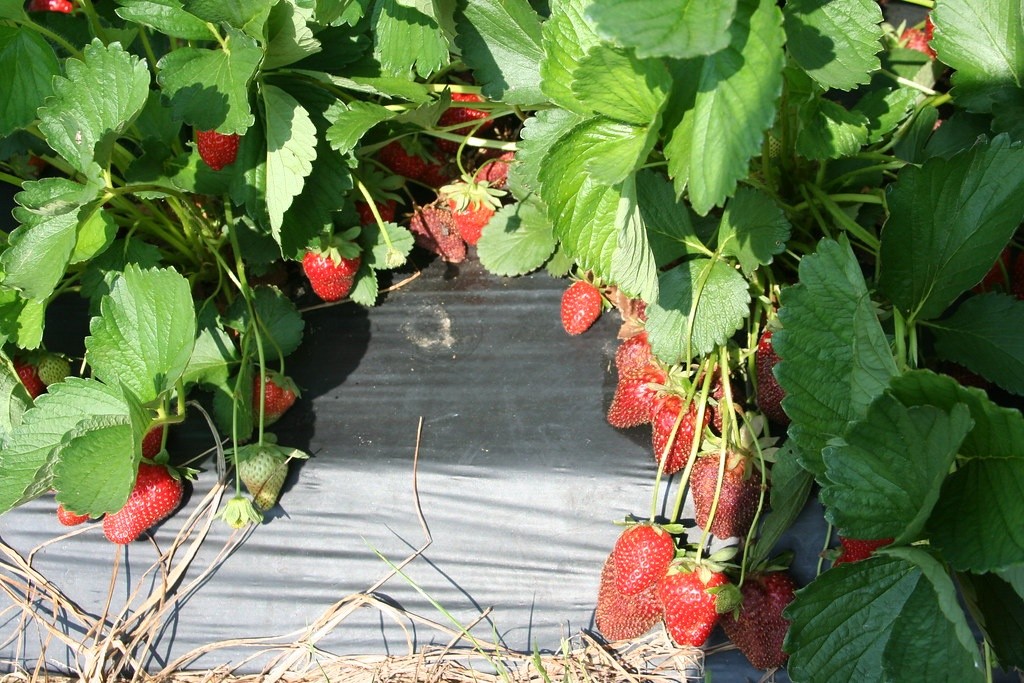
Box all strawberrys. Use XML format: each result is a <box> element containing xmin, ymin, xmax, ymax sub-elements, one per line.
<box><xmin>0</xmin><ymin>0</ymin><xmax>1024</xmax><ymax>670</ymax></box>
